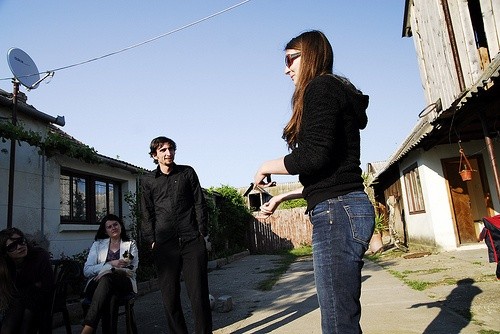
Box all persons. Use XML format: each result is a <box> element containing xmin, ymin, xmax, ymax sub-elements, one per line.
<box><xmin>136</xmin><ymin>136</ymin><xmax>212</xmax><ymax>334</ymax></box>
<box><xmin>80</xmin><ymin>214</ymin><xmax>140</xmax><ymax>334</ymax></box>
<box><xmin>0</xmin><ymin>227</ymin><xmax>56</xmax><ymax>334</ymax></box>
<box><xmin>252</xmin><ymin>30</ymin><xmax>375</xmax><ymax>334</ymax></box>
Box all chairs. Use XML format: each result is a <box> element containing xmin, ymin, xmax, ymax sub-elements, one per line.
<box><xmin>50</xmin><ymin>260</ymin><xmax>138</xmax><ymax>334</ymax></box>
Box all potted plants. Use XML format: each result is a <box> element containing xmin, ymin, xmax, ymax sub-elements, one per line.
<box><xmin>369</xmin><ymin>213</ymin><xmax>391</xmax><ymax>255</ymax></box>
<box><xmin>460</xmin><ymin>165</ymin><xmax>472</xmax><ymax>181</ymax></box>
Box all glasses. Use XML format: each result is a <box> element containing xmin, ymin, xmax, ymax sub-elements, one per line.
<box><xmin>284</xmin><ymin>51</ymin><xmax>303</xmax><ymax>68</ymax></box>
<box><xmin>2</xmin><ymin>237</ymin><xmax>27</xmax><ymax>253</ymax></box>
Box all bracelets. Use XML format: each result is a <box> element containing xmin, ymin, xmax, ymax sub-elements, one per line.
<box><xmin>208</xmin><ymin>238</ymin><xmax>212</xmax><ymax>243</ymax></box>
<box><xmin>111</xmin><ymin>267</ymin><xmax>116</xmax><ymax>273</ymax></box>
